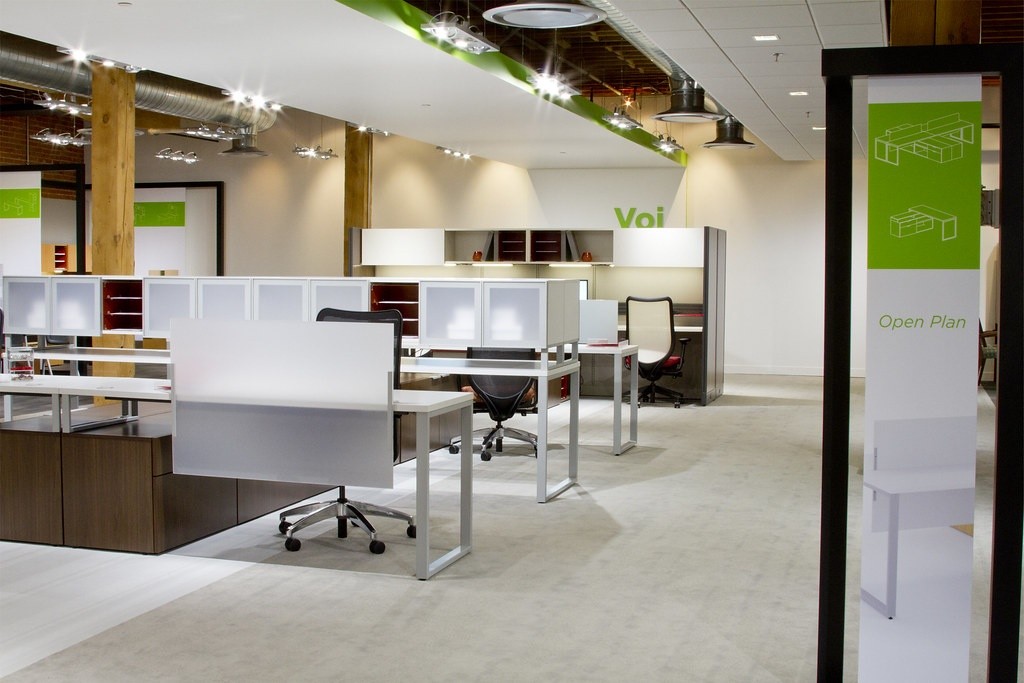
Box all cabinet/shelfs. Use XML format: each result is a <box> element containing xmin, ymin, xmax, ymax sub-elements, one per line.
<box><xmin>421</xmin><ymin>279</ymin><xmax>580</xmax><ymax>371</ymax></box>
<box><xmin>252</xmin><ymin>279</ymin><xmax>367</xmax><ymax>324</ymax></box>
<box><xmin>101</xmin><ymin>279</ymin><xmax>143</xmax><ymax>331</ymax></box>
<box><xmin>3</xmin><ymin>277</ymin><xmax>101</xmax><ymax>336</ymax></box>
<box><xmin>144</xmin><ymin>279</ymin><xmax>251</xmax><ymax>338</ymax></box>
<box><xmin>369</xmin><ymin>279</ymin><xmax>419</xmax><ymax>340</ymax></box>
<box><xmin>55</xmin><ymin>245</ymin><xmax>68</xmax><ymax>271</ymax></box>
<box><xmin>0</xmin><ymin>374</ymin><xmax>570</xmax><ymax>554</ymax></box>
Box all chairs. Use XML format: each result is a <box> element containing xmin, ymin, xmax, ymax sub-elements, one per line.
<box><xmin>277</xmin><ymin>309</ymin><xmax>416</xmax><ymax>554</ymax></box>
<box><xmin>622</xmin><ymin>294</ymin><xmax>684</xmax><ymax>408</ymax></box>
<box><xmin>450</xmin><ymin>347</ymin><xmax>537</xmax><ymax>462</ymax></box>
<box><xmin>978</xmin><ymin>318</ymin><xmax>998</xmax><ymax>388</ymax></box>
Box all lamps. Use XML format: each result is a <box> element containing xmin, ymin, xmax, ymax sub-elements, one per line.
<box><xmin>155</xmin><ymin>147</ymin><xmax>198</xmax><ymax>164</ymax></box>
<box><xmin>535</xmin><ymin>31</ymin><xmax>583</xmax><ymax>97</ymax></box>
<box><xmin>602</xmin><ymin>62</ymin><xmax>643</xmax><ymax>130</ymax></box>
<box><xmin>422</xmin><ymin>0</ymin><xmax>499</xmax><ymax>55</ymax></box>
<box><xmin>651</xmin><ymin>92</ymin><xmax>684</xmax><ymax>151</ymax></box>
<box><xmin>32</xmin><ymin>89</ymin><xmax>94</xmax><ymax>116</ymax></box>
<box><xmin>31</xmin><ymin>116</ymin><xmax>91</xmax><ymax>147</ymax></box>
<box><xmin>293</xmin><ymin>110</ymin><xmax>339</xmax><ymax>160</ymax></box>
<box><xmin>185</xmin><ymin>121</ymin><xmax>243</xmax><ymax>140</ymax></box>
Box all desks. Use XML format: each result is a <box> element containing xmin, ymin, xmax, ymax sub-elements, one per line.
<box><xmin>401</xmin><ymin>336</ymin><xmax>640</xmax><ymax>456</ymax></box>
<box><xmin>618</xmin><ymin>326</ymin><xmax>703</xmax><ymax>332</ymax></box>
<box><xmin>0</xmin><ymin>371</ymin><xmax>474</xmax><ymax>581</ymax></box>
